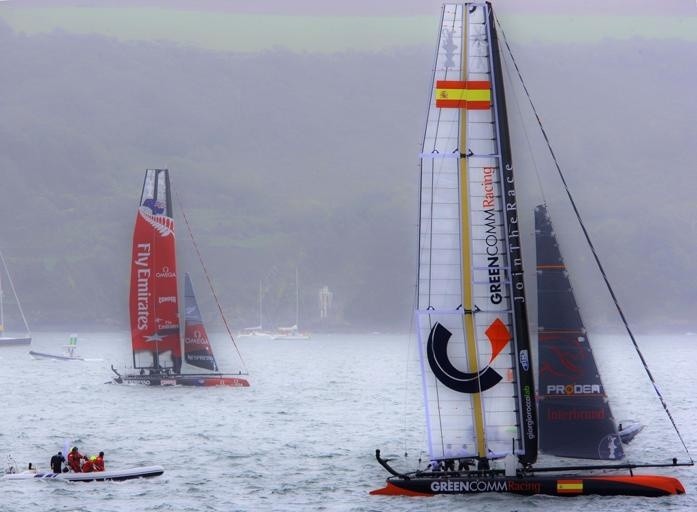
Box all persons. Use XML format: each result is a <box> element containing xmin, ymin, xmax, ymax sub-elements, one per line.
<box><xmin>50</xmin><ymin>447</ymin><xmax>105</xmax><ymax>472</ymax></box>
<box><xmin>140</xmin><ymin>368</ymin><xmax>145</xmax><ymax>375</ymax></box>
<box><xmin>424</xmin><ymin>441</ymin><xmax>494</xmax><ymax>476</ymax></box>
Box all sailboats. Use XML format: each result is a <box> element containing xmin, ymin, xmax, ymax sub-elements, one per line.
<box><xmin>369</xmin><ymin>0</ymin><xmax>695</xmax><ymax>497</ymax></box>
<box><xmin>111</xmin><ymin>168</ymin><xmax>251</xmax><ymax>388</ymax></box>
<box><xmin>0</xmin><ymin>254</ymin><xmax>31</xmax><ymax>346</ymax></box>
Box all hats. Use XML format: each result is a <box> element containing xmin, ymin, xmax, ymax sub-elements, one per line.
<box><xmin>91</xmin><ymin>456</ymin><xmax>96</xmax><ymax>461</ymax></box>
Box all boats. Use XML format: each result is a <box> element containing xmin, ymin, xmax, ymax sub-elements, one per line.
<box><xmin>29</xmin><ymin>336</ymin><xmax>84</xmax><ymax>362</ymax></box>
<box><xmin>236</xmin><ymin>280</ymin><xmax>273</xmax><ymax>338</ymax></box>
<box><xmin>4</xmin><ymin>464</ymin><xmax>163</xmax><ymax>482</ymax></box>
<box><xmin>270</xmin><ymin>269</ymin><xmax>309</xmax><ymax>340</ymax></box>
